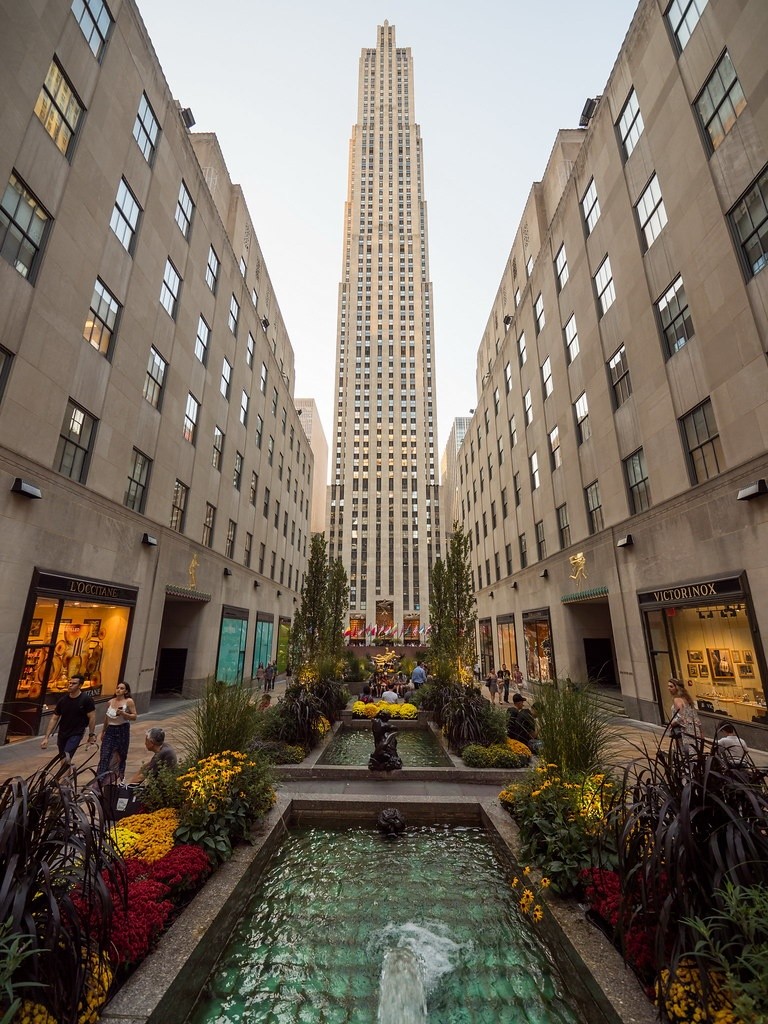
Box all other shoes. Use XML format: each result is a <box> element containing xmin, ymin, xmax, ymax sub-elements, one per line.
<box><xmin>262</xmin><ymin>690</ymin><xmax>266</xmax><ymax>694</ymax></box>
<box><xmin>118</xmin><ymin>777</ymin><xmax>127</xmax><ymax>787</ymax></box>
<box><xmin>499</xmin><ymin>701</ymin><xmax>503</xmax><ymax>704</ymax></box>
<box><xmin>88</xmin><ymin>790</ymin><xmax>101</xmax><ymax>807</ymax></box>
<box><xmin>491</xmin><ymin>702</ymin><xmax>496</xmax><ymax>706</ymax></box>
<box><xmin>266</xmin><ymin>688</ymin><xmax>275</xmax><ymax>693</ymax></box>
<box><xmin>504</xmin><ymin>700</ymin><xmax>509</xmax><ymax>703</ymax></box>
<box><xmin>257</xmin><ymin>688</ymin><xmax>259</xmax><ymax>692</ymax></box>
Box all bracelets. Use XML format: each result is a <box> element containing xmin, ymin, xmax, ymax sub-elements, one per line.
<box><xmin>89</xmin><ymin>733</ymin><xmax>95</xmax><ymax>736</ymax></box>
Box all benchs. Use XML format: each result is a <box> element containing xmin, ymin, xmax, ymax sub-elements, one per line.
<box><xmin>20</xmin><ymin>693</ymin><xmax>116</xmax><ymax>736</ymax></box>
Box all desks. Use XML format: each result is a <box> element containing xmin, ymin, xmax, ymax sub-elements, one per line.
<box><xmin>696</xmin><ymin>687</ymin><xmax>768</xmax><ymax>724</ymax></box>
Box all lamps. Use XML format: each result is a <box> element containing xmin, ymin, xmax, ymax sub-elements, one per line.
<box><xmin>540</xmin><ymin>569</ymin><xmax>548</xmax><ymax>577</ymax></box>
<box><xmin>737</xmin><ymin>478</ymin><xmax>768</xmax><ymax>501</ymax></box>
<box><xmin>617</xmin><ymin>534</ymin><xmax>634</xmax><ymax>548</ymax></box>
<box><xmin>488</xmin><ymin>591</ymin><xmax>493</xmax><ymax>596</ymax></box>
<box><xmin>141</xmin><ymin>533</ymin><xmax>158</xmax><ymax>546</ymax></box>
<box><xmin>254</xmin><ymin>580</ymin><xmax>260</xmax><ymax>586</ymax></box>
<box><xmin>695</xmin><ymin>603</ymin><xmax>747</xmax><ymax>619</ymax></box>
<box><xmin>510</xmin><ymin>581</ymin><xmax>517</xmax><ymax>589</ymax></box>
<box><xmin>225</xmin><ymin>567</ymin><xmax>232</xmax><ymax>576</ymax></box>
<box><xmin>277</xmin><ymin>590</ymin><xmax>282</xmax><ymax>598</ymax></box>
<box><xmin>10</xmin><ymin>477</ymin><xmax>43</xmax><ymax>500</ymax></box>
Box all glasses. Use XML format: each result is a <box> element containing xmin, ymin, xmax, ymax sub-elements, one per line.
<box><xmin>69</xmin><ymin>682</ymin><xmax>78</xmax><ymax>685</ymax></box>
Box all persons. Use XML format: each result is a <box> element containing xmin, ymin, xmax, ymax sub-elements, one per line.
<box><xmin>382</xmin><ymin>684</ymin><xmax>398</xmax><ymax>703</ymax></box>
<box><xmin>369</xmin><ymin>671</ymin><xmax>407</xmax><ymax>698</ymax></box>
<box><xmin>506</xmin><ymin>693</ymin><xmax>526</xmax><ymax>731</ymax></box>
<box><xmin>256</xmin><ymin>659</ymin><xmax>294</xmax><ymax>693</ymax></box>
<box><xmin>86</xmin><ymin>682</ymin><xmax>137</xmax><ymax>797</ymax></box>
<box><xmin>359</xmin><ymin>686</ymin><xmax>374</xmax><ymax>703</ymax></box>
<box><xmin>566</xmin><ymin>678</ymin><xmax>578</xmax><ymax>692</ymax></box>
<box><xmin>506</xmin><ymin>703</ymin><xmax>545</xmax><ymax>756</ymax></box>
<box><xmin>487</xmin><ymin>664</ymin><xmax>523</xmax><ymax>705</ymax></box>
<box><xmin>715</xmin><ymin>721</ymin><xmax>748</xmax><ymax>767</ymax></box>
<box><xmin>466</xmin><ymin>661</ymin><xmax>479</xmax><ymax>681</ymax></box>
<box><xmin>129</xmin><ymin>728</ymin><xmax>177</xmax><ymax>783</ymax></box>
<box><xmin>412</xmin><ymin>660</ymin><xmax>427</xmax><ymax>689</ymax></box>
<box><xmin>668</xmin><ymin>678</ymin><xmax>702</xmax><ymax>768</ymax></box>
<box><xmin>41</xmin><ymin>675</ymin><xmax>95</xmax><ymax>794</ymax></box>
<box><xmin>258</xmin><ymin>694</ymin><xmax>272</xmax><ymax>711</ymax></box>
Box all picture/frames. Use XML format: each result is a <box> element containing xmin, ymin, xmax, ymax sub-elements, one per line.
<box><xmin>685</xmin><ymin>648</ymin><xmax>756</xmax><ymax>679</ymax></box>
<box><xmin>28</xmin><ymin>615</ymin><xmax>102</xmax><ymax>637</ymax></box>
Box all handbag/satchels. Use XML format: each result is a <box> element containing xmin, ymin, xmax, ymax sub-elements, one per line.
<box><xmin>484</xmin><ymin>673</ymin><xmax>491</xmax><ymax>687</ymax></box>
<box><xmin>101</xmin><ymin>780</ymin><xmax>153</xmax><ymax>821</ymax></box>
<box><xmin>517</xmin><ymin>683</ymin><xmax>523</xmax><ymax>689</ymax></box>
<box><xmin>668</xmin><ymin>725</ymin><xmax>682</xmax><ymax>740</ymax></box>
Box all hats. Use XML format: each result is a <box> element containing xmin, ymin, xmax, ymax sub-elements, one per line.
<box><xmin>513</xmin><ymin>693</ymin><xmax>526</xmax><ymax>702</ymax></box>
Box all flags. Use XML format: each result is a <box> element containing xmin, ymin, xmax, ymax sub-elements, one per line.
<box><xmin>383</xmin><ymin>624</ymin><xmax>398</xmax><ymax>636</ymax></box>
<box><xmin>368</xmin><ymin>624</ymin><xmax>377</xmax><ymax>636</ymax></box>
<box><xmin>358</xmin><ymin>625</ymin><xmax>370</xmax><ymax>635</ymax></box>
<box><xmin>399</xmin><ymin>624</ymin><xmax>411</xmax><ymax>638</ymax></box>
<box><xmin>377</xmin><ymin>625</ymin><xmax>384</xmax><ymax>637</ymax></box>
<box><xmin>343</xmin><ymin>626</ymin><xmax>350</xmax><ymax>636</ymax></box>
<box><xmin>413</xmin><ymin>624</ymin><xmax>431</xmax><ymax>636</ymax></box>
<box><xmin>352</xmin><ymin>626</ymin><xmax>357</xmax><ymax>636</ymax></box>
